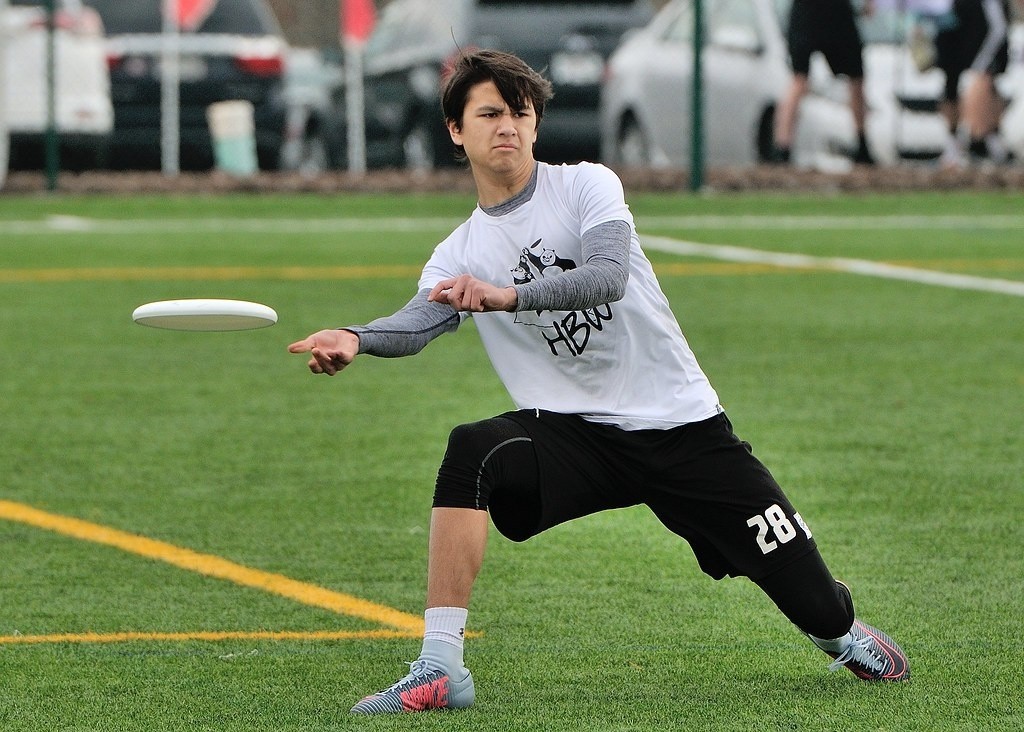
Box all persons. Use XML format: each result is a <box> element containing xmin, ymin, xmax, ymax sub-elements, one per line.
<box><xmin>288</xmin><ymin>51</ymin><xmax>910</xmax><ymax>713</ymax></box>
<box><xmin>769</xmin><ymin>0</ymin><xmax>879</xmax><ymax>172</ymax></box>
<box><xmin>911</xmin><ymin>0</ymin><xmax>1019</xmax><ymax>178</ymax></box>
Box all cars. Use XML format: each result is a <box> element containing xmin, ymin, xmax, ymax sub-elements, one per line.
<box><xmin>595</xmin><ymin>0</ymin><xmax>954</xmax><ymax>172</ymax></box>
<box><xmin>81</xmin><ymin>0</ymin><xmax>292</xmax><ymax>170</ymax></box>
<box><xmin>317</xmin><ymin>0</ymin><xmax>657</xmax><ymax>171</ymax></box>
<box><xmin>0</xmin><ymin>0</ymin><xmax>115</xmax><ymax>136</ymax></box>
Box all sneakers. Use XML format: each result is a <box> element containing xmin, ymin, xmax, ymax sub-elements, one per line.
<box><xmin>350</xmin><ymin>660</ymin><xmax>476</xmax><ymax>715</ymax></box>
<box><xmin>794</xmin><ymin>618</ymin><xmax>911</xmax><ymax>682</ymax></box>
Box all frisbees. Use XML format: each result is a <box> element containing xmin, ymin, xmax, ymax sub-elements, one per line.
<box><xmin>130</xmin><ymin>298</ymin><xmax>279</xmax><ymax>330</ymax></box>
